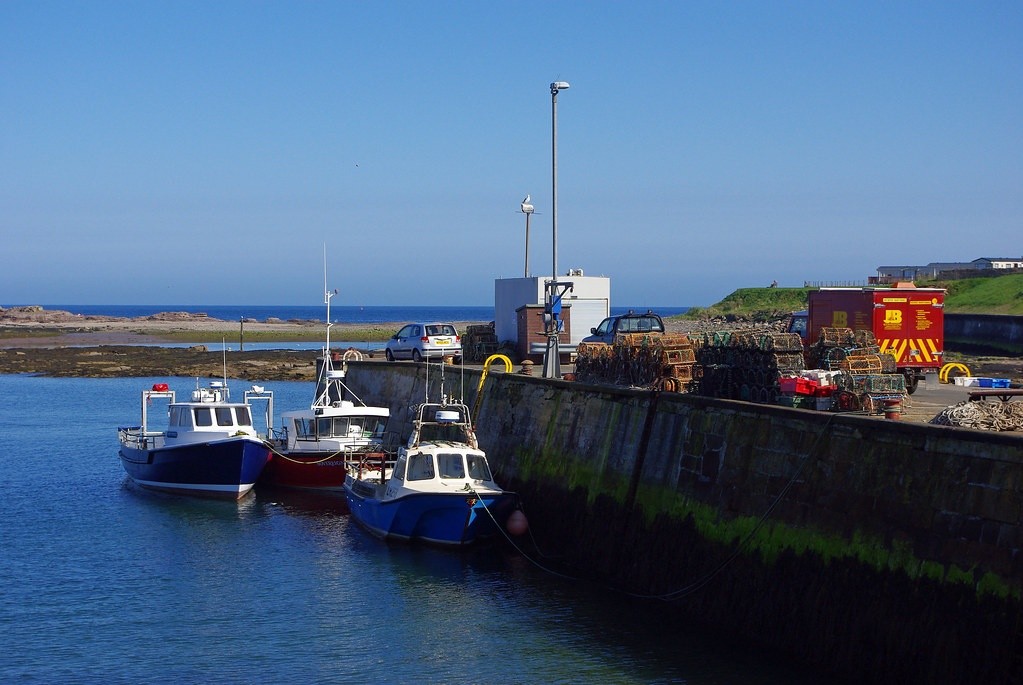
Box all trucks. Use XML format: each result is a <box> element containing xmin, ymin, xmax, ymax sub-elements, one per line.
<box><xmin>780</xmin><ymin>282</ymin><xmax>947</xmax><ymax>396</ymax></box>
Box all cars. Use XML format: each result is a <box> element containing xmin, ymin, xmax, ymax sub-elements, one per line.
<box><xmin>385</xmin><ymin>321</ymin><xmax>463</xmax><ymax>362</ymax></box>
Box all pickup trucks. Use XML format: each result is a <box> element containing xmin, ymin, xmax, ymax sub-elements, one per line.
<box><xmin>581</xmin><ymin>310</ymin><xmax>666</xmax><ymax>345</ymax></box>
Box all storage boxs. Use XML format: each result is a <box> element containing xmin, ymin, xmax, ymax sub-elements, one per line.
<box><xmin>774</xmin><ymin>368</ymin><xmax>846</xmax><ymax>411</ymax></box>
<box><xmin>954</xmin><ymin>377</ymin><xmax>1012</xmax><ymax>389</ymax></box>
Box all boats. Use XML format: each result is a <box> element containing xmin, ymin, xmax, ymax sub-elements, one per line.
<box><xmin>117</xmin><ymin>334</ymin><xmax>275</xmax><ymax>499</ymax></box>
<box><xmin>340</xmin><ymin>350</ymin><xmax>522</xmax><ymax>552</ymax></box>
<box><xmin>265</xmin><ymin>241</ymin><xmax>400</xmax><ymax>497</ymax></box>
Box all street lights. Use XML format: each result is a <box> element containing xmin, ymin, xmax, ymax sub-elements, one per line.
<box><xmin>521</xmin><ymin>195</ymin><xmax>535</xmax><ymax>278</ymax></box>
<box><xmin>550</xmin><ymin>81</ymin><xmax>572</xmax><ymax>296</ymax></box>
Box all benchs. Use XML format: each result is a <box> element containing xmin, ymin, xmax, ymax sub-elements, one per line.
<box><xmin>967</xmin><ymin>388</ymin><xmax>1023</xmax><ymax>401</ymax></box>
